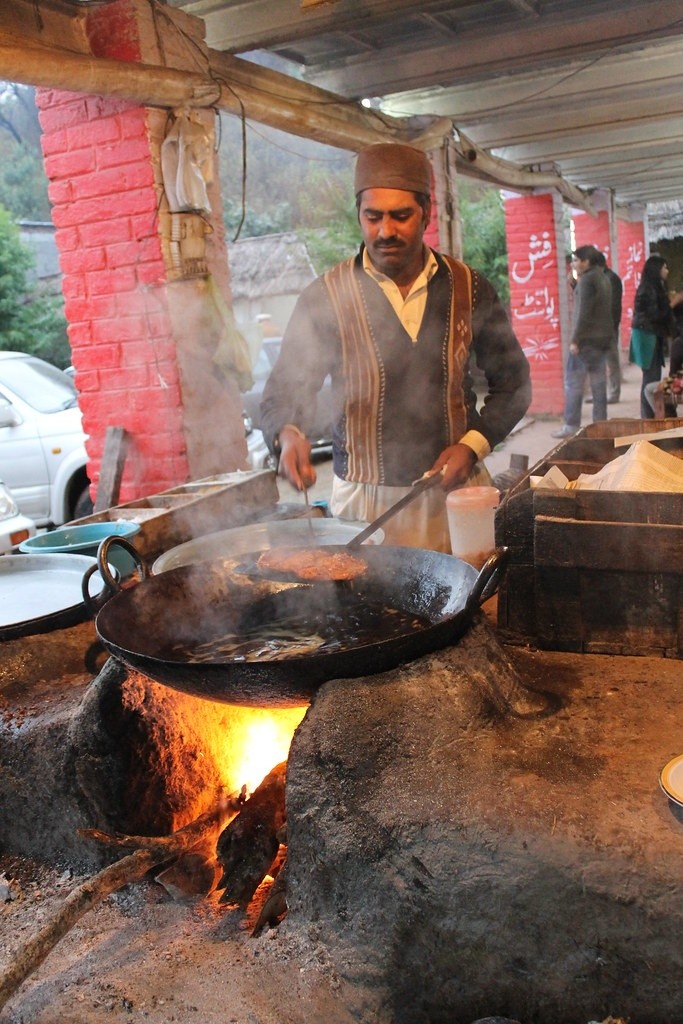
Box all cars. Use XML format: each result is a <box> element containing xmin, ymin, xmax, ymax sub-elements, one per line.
<box><xmin>242</xmin><ymin>337</ymin><xmax>333</xmax><ymax>470</ymax></box>
<box><xmin>0</xmin><ymin>351</ymin><xmax>94</xmax><ymax>557</ymax></box>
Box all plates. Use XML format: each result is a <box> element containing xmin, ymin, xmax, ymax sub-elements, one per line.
<box><xmin>659</xmin><ymin>755</ymin><xmax>683</xmax><ymax>807</ymax></box>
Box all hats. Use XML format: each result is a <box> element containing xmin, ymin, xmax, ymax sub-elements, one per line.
<box><xmin>354</xmin><ymin>143</ymin><xmax>432</xmax><ymax>196</ymax></box>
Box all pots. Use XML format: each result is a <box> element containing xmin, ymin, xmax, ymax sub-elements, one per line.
<box><xmin>96</xmin><ymin>535</ymin><xmax>509</xmax><ymax>708</ymax></box>
<box><xmin>0</xmin><ymin>553</ymin><xmax>122</xmax><ymax>642</ymax></box>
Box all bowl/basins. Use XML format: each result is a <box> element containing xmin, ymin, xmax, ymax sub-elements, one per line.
<box><xmin>19</xmin><ymin>522</ymin><xmax>141</xmax><ymax>582</ymax></box>
<box><xmin>152</xmin><ymin>518</ymin><xmax>386</xmax><ymax>576</ymax></box>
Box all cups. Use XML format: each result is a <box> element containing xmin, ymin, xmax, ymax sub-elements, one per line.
<box><xmin>446</xmin><ymin>486</ymin><xmax>502</xmax><ymax>572</ymax></box>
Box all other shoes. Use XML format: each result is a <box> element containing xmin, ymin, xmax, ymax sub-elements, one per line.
<box><xmin>583</xmin><ymin>394</ymin><xmax>600</xmax><ymax>404</ymax></box>
<box><xmin>551</xmin><ymin>424</ymin><xmax>580</xmax><ymax>438</ymax></box>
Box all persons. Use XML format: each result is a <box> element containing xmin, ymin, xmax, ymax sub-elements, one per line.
<box><xmin>628</xmin><ymin>254</ymin><xmax>683</xmax><ymax>418</ymax></box>
<box><xmin>261</xmin><ymin>144</ymin><xmax>534</xmax><ymax>561</ymax></box>
<box><xmin>551</xmin><ymin>244</ymin><xmax>625</xmax><ymax>437</ymax></box>
